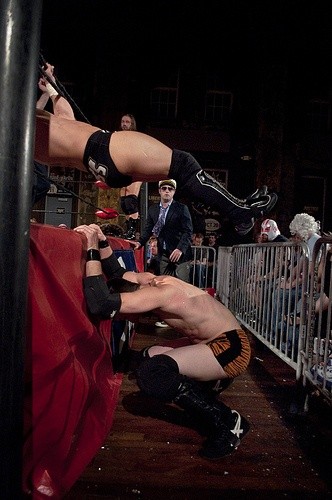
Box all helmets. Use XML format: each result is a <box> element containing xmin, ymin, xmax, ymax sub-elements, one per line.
<box><xmin>261</xmin><ymin>219</ymin><xmax>280</xmax><ymax>241</ymax></box>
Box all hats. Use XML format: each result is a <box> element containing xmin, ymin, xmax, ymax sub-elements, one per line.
<box><xmin>159</xmin><ymin>178</ymin><xmax>177</xmax><ymax>188</ymax></box>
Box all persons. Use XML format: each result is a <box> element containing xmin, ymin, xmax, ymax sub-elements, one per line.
<box><xmin>34</xmin><ymin>63</ymin><xmax>278</xmax><ymax>237</ymax></box>
<box><xmin>73</xmin><ymin>223</ymin><xmax>250</xmax><ymax>459</ymax></box>
<box><xmin>119</xmin><ymin>115</ymin><xmax>142</xmax><ymax>239</ymax></box>
<box><xmin>136</xmin><ymin>213</ymin><xmax>332</xmax><ymax>384</ymax></box>
<box><xmin>123</xmin><ymin>179</ymin><xmax>193</xmax><ymax>328</ymax></box>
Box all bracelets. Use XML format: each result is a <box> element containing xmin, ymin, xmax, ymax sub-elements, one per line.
<box><xmin>85</xmin><ymin>249</ymin><xmax>101</xmax><ymax>262</ymax></box>
<box><xmin>98</xmin><ymin>240</ymin><xmax>109</xmax><ymax>248</ymax></box>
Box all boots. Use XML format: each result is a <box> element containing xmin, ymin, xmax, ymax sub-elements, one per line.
<box><xmin>123</xmin><ymin>217</ymin><xmax>137</xmax><ymax>239</ymax></box>
<box><xmin>175</xmin><ymin>377</ymin><xmax>251</xmax><ymax>458</ymax></box>
<box><xmin>182</xmin><ymin>170</ymin><xmax>278</xmax><ymax>236</ymax></box>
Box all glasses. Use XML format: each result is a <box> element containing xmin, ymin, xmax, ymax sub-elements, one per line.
<box><xmin>162</xmin><ymin>186</ymin><xmax>174</xmax><ymax>191</ymax></box>
